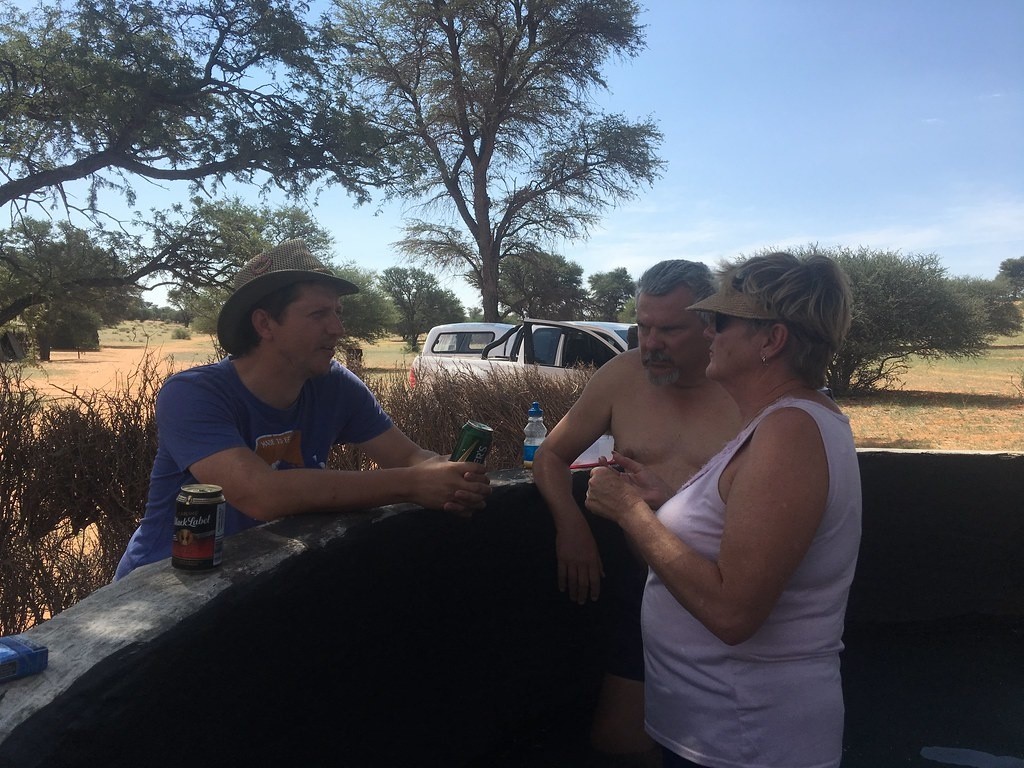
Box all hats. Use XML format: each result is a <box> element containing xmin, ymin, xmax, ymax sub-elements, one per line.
<box><xmin>685</xmin><ymin>286</ymin><xmax>838</xmax><ymax>352</ymax></box>
<box><xmin>218</xmin><ymin>238</ymin><xmax>360</xmax><ymax>355</ymax></box>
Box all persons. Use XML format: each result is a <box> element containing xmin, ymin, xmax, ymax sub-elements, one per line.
<box><xmin>114</xmin><ymin>238</ymin><xmax>496</xmax><ymax>582</ymax></box>
<box><xmin>582</xmin><ymin>252</ymin><xmax>865</xmax><ymax>766</ymax></box>
<box><xmin>533</xmin><ymin>258</ymin><xmax>754</xmax><ymax>768</ymax></box>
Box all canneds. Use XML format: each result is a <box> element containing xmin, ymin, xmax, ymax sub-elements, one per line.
<box><xmin>171</xmin><ymin>485</ymin><xmax>227</xmax><ymax>573</ymax></box>
<box><xmin>448</xmin><ymin>419</ymin><xmax>494</xmax><ymax>465</ymax></box>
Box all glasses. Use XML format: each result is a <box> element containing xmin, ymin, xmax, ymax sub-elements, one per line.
<box><xmin>715</xmin><ymin>312</ymin><xmax>801</xmax><ymax>342</ymax></box>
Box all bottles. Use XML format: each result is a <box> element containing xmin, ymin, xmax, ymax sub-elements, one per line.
<box><xmin>524</xmin><ymin>400</ymin><xmax>548</xmax><ymax>468</ymax></box>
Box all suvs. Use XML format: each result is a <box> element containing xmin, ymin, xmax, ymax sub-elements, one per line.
<box><xmin>410</xmin><ymin>318</ymin><xmax>638</xmax><ymax>404</ymax></box>
